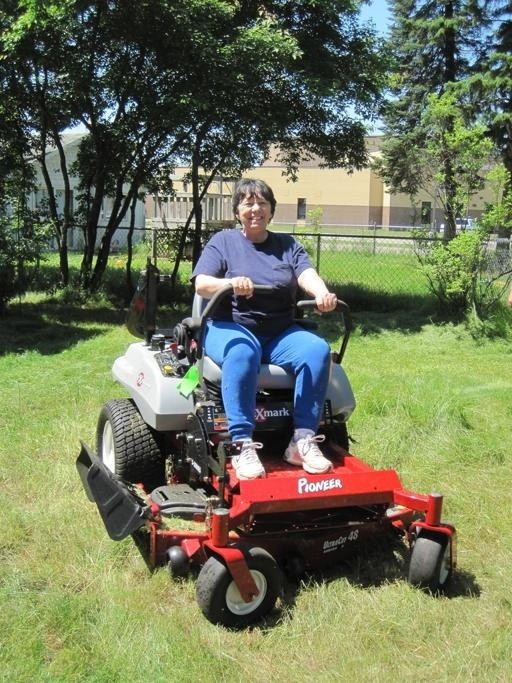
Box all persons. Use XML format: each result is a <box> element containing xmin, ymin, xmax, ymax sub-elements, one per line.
<box><xmin>186</xmin><ymin>177</ymin><xmax>338</xmax><ymax>483</ymax></box>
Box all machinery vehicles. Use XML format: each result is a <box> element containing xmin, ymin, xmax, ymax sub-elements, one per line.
<box><xmin>75</xmin><ymin>257</ymin><xmax>458</xmax><ymax>628</ymax></box>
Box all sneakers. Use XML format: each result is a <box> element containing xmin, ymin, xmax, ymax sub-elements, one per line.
<box><xmin>231</xmin><ymin>437</ymin><xmax>267</xmax><ymax>480</ymax></box>
<box><xmin>283</xmin><ymin>432</ymin><xmax>334</xmax><ymax>473</ymax></box>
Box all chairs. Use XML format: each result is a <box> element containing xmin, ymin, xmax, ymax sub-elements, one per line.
<box><xmin>189</xmin><ymin>285</ymin><xmax>355</xmax><ymax>466</ymax></box>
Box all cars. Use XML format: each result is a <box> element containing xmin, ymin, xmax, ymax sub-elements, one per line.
<box><xmin>438</xmin><ymin>217</ymin><xmax>475</xmax><ymax>232</ymax></box>
